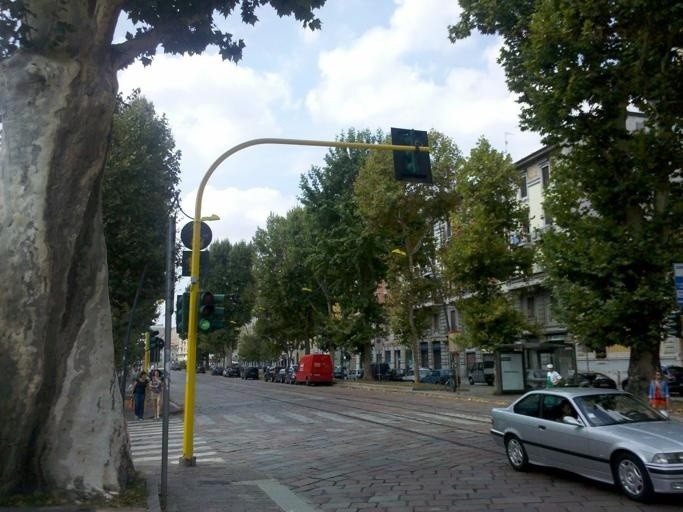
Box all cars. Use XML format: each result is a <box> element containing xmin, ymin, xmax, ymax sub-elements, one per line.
<box><xmin>333</xmin><ymin>362</ymin><xmax>462</xmax><ymax>393</ymax></box>
<box><xmin>487</xmin><ymin>365</ymin><xmax>683</xmax><ymax>502</ymax></box>
<box><xmin>170</xmin><ymin>361</ymin><xmax>299</xmax><ymax>386</ymax></box>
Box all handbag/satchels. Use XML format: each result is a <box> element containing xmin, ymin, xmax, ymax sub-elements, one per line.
<box><xmin>128</xmin><ymin>399</ymin><xmax>134</xmax><ymax>411</ymax></box>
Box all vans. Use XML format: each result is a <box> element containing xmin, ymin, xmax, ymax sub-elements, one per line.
<box><xmin>295</xmin><ymin>353</ymin><xmax>332</xmax><ymax>386</ymax></box>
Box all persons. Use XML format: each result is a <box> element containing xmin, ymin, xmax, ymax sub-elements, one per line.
<box><xmin>148</xmin><ymin>369</ymin><xmax>164</xmax><ymax>421</ymax></box>
<box><xmin>544</xmin><ymin>362</ymin><xmax>562</xmax><ymax>405</ymax></box>
<box><xmin>556</xmin><ymin>399</ymin><xmax>574</xmax><ymax>424</ymax></box>
<box><xmin>647</xmin><ymin>369</ymin><xmax>670</xmax><ymax>412</ymax></box>
<box><xmin>129</xmin><ymin>371</ymin><xmax>149</xmax><ymax>421</ymax></box>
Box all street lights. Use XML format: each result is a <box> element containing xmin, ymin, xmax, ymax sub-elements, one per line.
<box><xmin>119</xmin><ymin>189</ymin><xmax>221</xmax><ymax>410</ymax></box>
<box><xmin>301</xmin><ymin>287</ymin><xmax>323</xmax><ymax>355</ymax></box>
<box><xmin>391</xmin><ymin>249</ymin><xmax>451</xmax><ymax>333</ymax></box>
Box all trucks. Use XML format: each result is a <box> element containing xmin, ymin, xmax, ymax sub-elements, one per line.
<box><xmin>468</xmin><ymin>358</ymin><xmax>494</xmax><ymax>386</ymax></box>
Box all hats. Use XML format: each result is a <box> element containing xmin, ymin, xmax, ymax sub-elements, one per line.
<box><xmin>547</xmin><ymin>364</ymin><xmax>553</xmax><ymax>369</ymax></box>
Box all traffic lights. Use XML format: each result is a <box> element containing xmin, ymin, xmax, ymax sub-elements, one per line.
<box><xmin>149</xmin><ymin>330</ymin><xmax>158</xmax><ymax>351</ymax></box>
<box><xmin>196</xmin><ymin>290</ymin><xmax>216</xmax><ymax>334</ymax></box>
<box><xmin>211</xmin><ymin>293</ymin><xmax>225</xmax><ymax>332</ymax></box>
<box><xmin>176</xmin><ymin>295</ymin><xmax>184</xmax><ymax>333</ymax></box>
